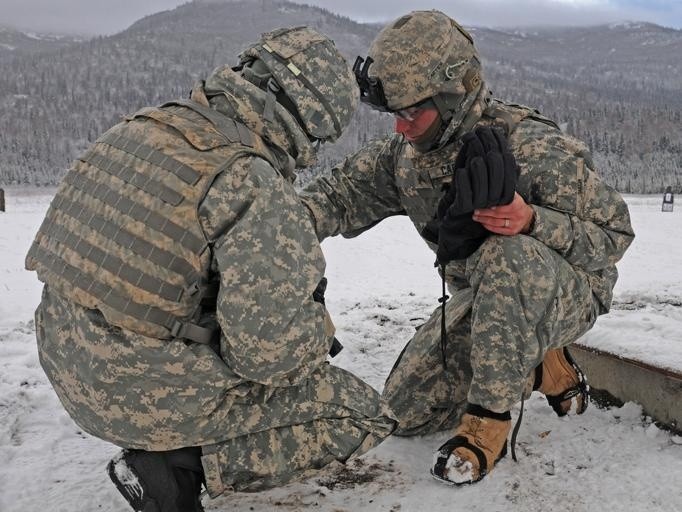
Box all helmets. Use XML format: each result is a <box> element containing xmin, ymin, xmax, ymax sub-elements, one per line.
<box><xmin>360</xmin><ymin>11</ymin><xmax>481</xmax><ymax>118</ymax></box>
<box><xmin>238</xmin><ymin>22</ymin><xmax>354</xmax><ymax>142</ymax></box>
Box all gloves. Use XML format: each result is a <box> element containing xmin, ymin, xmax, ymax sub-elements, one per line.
<box><xmin>421</xmin><ymin>122</ymin><xmax>520</xmax><ymax>269</ymax></box>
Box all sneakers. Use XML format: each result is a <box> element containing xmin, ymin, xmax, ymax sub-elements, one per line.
<box><xmin>103</xmin><ymin>445</ymin><xmax>212</xmax><ymax>512</ymax></box>
<box><xmin>520</xmin><ymin>339</ymin><xmax>587</xmax><ymax>420</ymax></box>
<box><xmin>430</xmin><ymin>401</ymin><xmax>515</xmax><ymax>487</ymax></box>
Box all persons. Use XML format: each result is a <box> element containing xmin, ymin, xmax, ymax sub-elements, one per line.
<box><xmin>300</xmin><ymin>10</ymin><xmax>636</xmax><ymax>488</ymax></box>
<box><xmin>21</xmin><ymin>26</ymin><xmax>399</xmax><ymax>512</ymax></box>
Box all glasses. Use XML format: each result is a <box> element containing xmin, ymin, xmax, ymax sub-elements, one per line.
<box><xmin>384</xmin><ymin>103</ymin><xmax>430</xmax><ymax>123</ymax></box>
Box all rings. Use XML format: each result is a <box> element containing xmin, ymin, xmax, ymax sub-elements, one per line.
<box><xmin>504</xmin><ymin>219</ymin><xmax>509</xmax><ymax>227</ymax></box>
